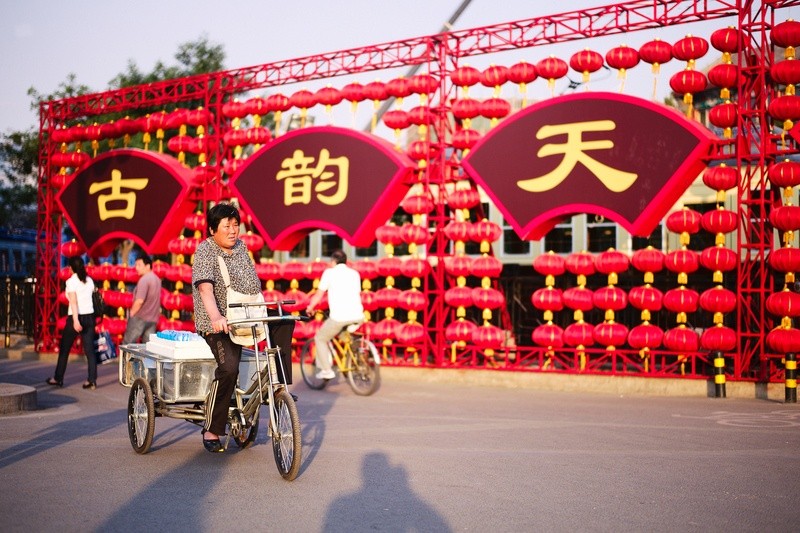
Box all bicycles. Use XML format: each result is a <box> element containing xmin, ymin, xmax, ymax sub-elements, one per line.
<box><xmin>300</xmin><ymin>309</ymin><xmax>381</xmax><ymax>398</ymax></box>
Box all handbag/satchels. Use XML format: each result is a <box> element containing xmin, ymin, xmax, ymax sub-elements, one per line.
<box><xmin>226</xmin><ymin>287</ymin><xmax>267</xmax><ymax>346</ymax></box>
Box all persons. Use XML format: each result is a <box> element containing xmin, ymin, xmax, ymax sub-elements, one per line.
<box><xmin>192</xmin><ymin>204</ymin><xmax>300</xmax><ymax>450</ymax></box>
<box><xmin>122</xmin><ymin>254</ymin><xmax>161</xmax><ymax>345</ymax></box>
<box><xmin>305</xmin><ymin>249</ymin><xmax>364</xmax><ymax>379</ymax></box>
<box><xmin>47</xmin><ymin>256</ymin><xmax>99</xmax><ymax>390</ymax></box>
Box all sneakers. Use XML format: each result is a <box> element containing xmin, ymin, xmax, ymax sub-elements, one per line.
<box><xmin>315</xmin><ymin>370</ymin><xmax>336</xmax><ymax>379</ymax></box>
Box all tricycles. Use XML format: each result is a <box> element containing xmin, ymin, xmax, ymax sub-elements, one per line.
<box><xmin>118</xmin><ymin>300</ymin><xmax>310</xmax><ymax>482</ymax></box>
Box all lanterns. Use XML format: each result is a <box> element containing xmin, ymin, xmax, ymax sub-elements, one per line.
<box><xmin>52</xmin><ymin>18</ymin><xmax>800</xmax><ymax>359</ymax></box>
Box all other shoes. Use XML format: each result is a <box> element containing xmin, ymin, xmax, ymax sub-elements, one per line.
<box><xmin>46</xmin><ymin>378</ymin><xmax>64</xmax><ymax>388</ymax></box>
<box><xmin>203</xmin><ymin>429</ymin><xmax>225</xmax><ymax>454</ymax></box>
<box><xmin>264</xmin><ymin>390</ymin><xmax>299</xmax><ymax>406</ymax></box>
<box><xmin>83</xmin><ymin>382</ymin><xmax>97</xmax><ymax>390</ymax></box>
